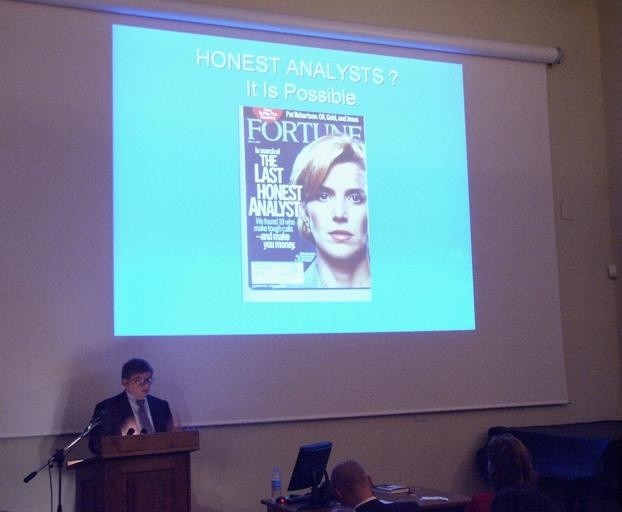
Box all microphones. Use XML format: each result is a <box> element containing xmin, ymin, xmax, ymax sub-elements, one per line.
<box><xmin>86</xmin><ymin>402</ymin><xmax>109</xmax><ymax>429</ymax></box>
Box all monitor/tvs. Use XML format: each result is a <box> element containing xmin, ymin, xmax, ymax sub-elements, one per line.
<box><xmin>287</xmin><ymin>440</ymin><xmax>335</xmax><ymax>505</ymax></box>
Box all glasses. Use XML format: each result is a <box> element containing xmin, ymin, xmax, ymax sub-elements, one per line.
<box><xmin>131</xmin><ymin>375</ymin><xmax>153</xmax><ymax>384</ymax></box>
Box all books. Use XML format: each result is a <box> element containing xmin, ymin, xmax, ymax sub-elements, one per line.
<box><xmin>372</xmin><ymin>484</ymin><xmax>411</xmax><ymax>496</ymax></box>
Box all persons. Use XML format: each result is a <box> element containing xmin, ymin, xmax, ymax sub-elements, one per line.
<box><xmin>465</xmin><ymin>434</ymin><xmax>556</xmax><ymax>512</ymax></box>
<box><xmin>330</xmin><ymin>459</ymin><xmax>421</xmax><ymax>512</ymax></box>
<box><xmin>280</xmin><ymin>133</ymin><xmax>370</xmax><ymax>288</ymax></box>
<box><xmin>88</xmin><ymin>359</ymin><xmax>174</xmax><ymax>456</ymax></box>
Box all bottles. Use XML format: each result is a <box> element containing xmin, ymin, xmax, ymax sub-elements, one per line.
<box><xmin>271</xmin><ymin>465</ymin><xmax>281</xmax><ymax>502</ymax></box>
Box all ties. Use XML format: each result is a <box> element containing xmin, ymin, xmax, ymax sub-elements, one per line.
<box><xmin>136</xmin><ymin>400</ymin><xmax>153</xmax><ymax>434</ymax></box>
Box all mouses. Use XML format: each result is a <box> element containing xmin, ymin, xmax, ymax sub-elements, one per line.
<box><xmin>275</xmin><ymin>494</ymin><xmax>287</xmax><ymax>506</ymax></box>
